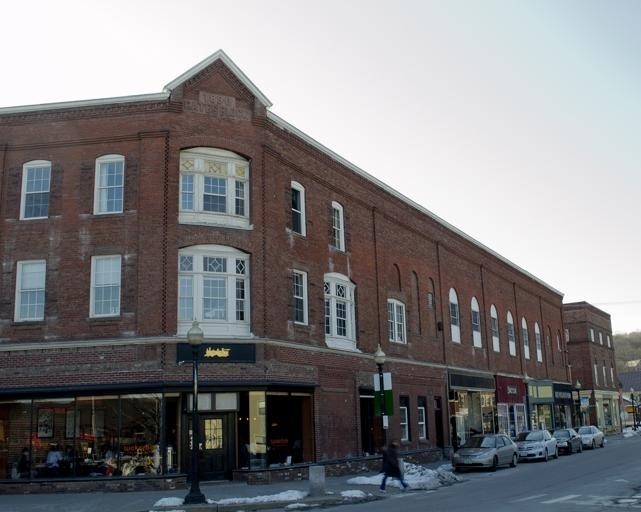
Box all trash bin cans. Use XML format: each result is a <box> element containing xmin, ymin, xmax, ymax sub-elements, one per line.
<box><xmin>165</xmin><ymin>447</ymin><xmax>174</xmax><ymax>471</ymax></box>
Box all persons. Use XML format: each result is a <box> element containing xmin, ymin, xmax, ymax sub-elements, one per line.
<box><xmin>19</xmin><ymin>448</ymin><xmax>30</xmax><ymax>478</ymax></box>
<box><xmin>378</xmin><ymin>441</ymin><xmax>410</xmax><ymax>492</ymax></box>
<box><xmin>47</xmin><ymin>445</ymin><xmax>78</xmax><ymax>468</ymax></box>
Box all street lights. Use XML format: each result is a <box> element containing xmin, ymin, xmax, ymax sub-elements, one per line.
<box><xmin>575</xmin><ymin>380</ymin><xmax>582</xmax><ymax>426</ymax></box>
<box><xmin>183</xmin><ymin>320</ymin><xmax>208</xmax><ymax>503</ymax></box>
<box><xmin>374</xmin><ymin>344</ymin><xmax>386</xmax><ymax>463</ymax></box>
<box><xmin>630</xmin><ymin>387</ymin><xmax>636</xmax><ymax>431</ymax></box>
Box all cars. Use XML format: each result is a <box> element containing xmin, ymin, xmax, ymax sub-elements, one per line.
<box><xmin>452</xmin><ymin>425</ymin><xmax>604</xmax><ymax>472</ymax></box>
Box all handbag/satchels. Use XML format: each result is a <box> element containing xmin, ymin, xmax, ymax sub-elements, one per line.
<box><xmin>397</xmin><ymin>458</ymin><xmax>405</xmax><ymax>478</ymax></box>
